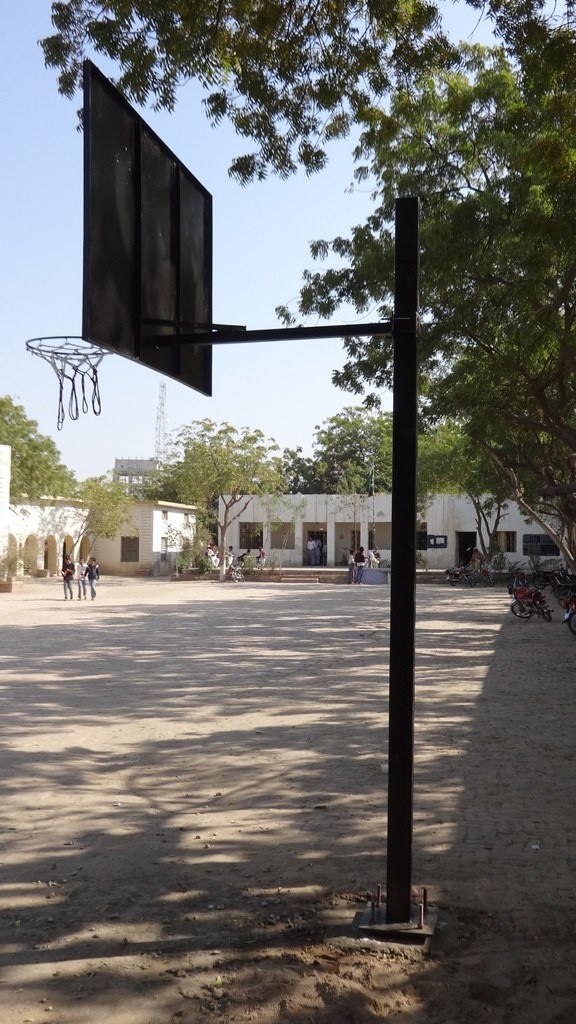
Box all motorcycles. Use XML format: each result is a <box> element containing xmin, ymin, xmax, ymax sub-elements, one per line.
<box><xmin>444</xmin><ymin>545</ymin><xmax>576</xmax><ymax>635</ymax></box>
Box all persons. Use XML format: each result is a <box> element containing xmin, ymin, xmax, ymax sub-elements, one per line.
<box><xmin>355</xmin><ymin>547</ymin><xmax>365</xmax><ymax>584</ymax></box>
<box><xmin>313</xmin><ymin>536</ymin><xmax>322</xmax><ymax>566</ymax></box>
<box><xmin>61</xmin><ymin>555</ymin><xmax>75</xmax><ymax>600</ymax></box>
<box><xmin>83</xmin><ymin>558</ymin><xmax>99</xmax><ymax>601</ymax></box>
<box><xmin>204</xmin><ymin>543</ymin><xmax>266</xmax><ymax>570</ymax></box>
<box><xmin>362</xmin><ymin>546</ymin><xmax>380</xmax><ymax>568</ymax></box>
<box><xmin>470</xmin><ymin>548</ymin><xmax>484</xmax><ymax>572</ymax></box>
<box><xmin>306</xmin><ymin>537</ymin><xmax>317</xmax><ymax>567</ymax></box>
<box><xmin>75</xmin><ymin>557</ymin><xmax>87</xmax><ymax>601</ymax></box>
<box><xmin>348</xmin><ymin>551</ymin><xmax>355</xmax><ymax>584</ymax></box>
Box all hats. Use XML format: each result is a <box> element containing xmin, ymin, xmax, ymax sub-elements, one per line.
<box><xmin>64</xmin><ymin>555</ymin><xmax>70</xmax><ymax>559</ymax></box>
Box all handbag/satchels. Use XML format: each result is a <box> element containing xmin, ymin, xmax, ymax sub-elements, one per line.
<box><xmin>357</xmin><ymin>562</ymin><xmax>365</xmax><ymax>567</ymax></box>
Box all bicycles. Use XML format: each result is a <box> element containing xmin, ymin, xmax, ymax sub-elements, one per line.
<box><xmin>229</xmin><ymin>564</ymin><xmax>245</xmax><ymax>583</ymax></box>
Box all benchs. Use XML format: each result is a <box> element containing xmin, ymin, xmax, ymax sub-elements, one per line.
<box><xmin>170</xmin><ymin>567</ymin><xmax>200</xmax><ymax>583</ymax></box>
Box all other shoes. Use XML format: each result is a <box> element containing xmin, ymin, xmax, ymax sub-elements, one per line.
<box><xmin>70</xmin><ymin>596</ymin><xmax>73</xmax><ymax>599</ymax></box>
<box><xmin>77</xmin><ymin>598</ymin><xmax>81</xmax><ymax>601</ymax></box>
<box><xmin>84</xmin><ymin>597</ymin><xmax>87</xmax><ymax>600</ymax></box>
<box><xmin>349</xmin><ymin>581</ymin><xmax>362</xmax><ymax>585</ymax></box>
<box><xmin>91</xmin><ymin>596</ymin><xmax>95</xmax><ymax>601</ymax></box>
<box><xmin>64</xmin><ymin>597</ymin><xmax>68</xmax><ymax>601</ymax></box>
